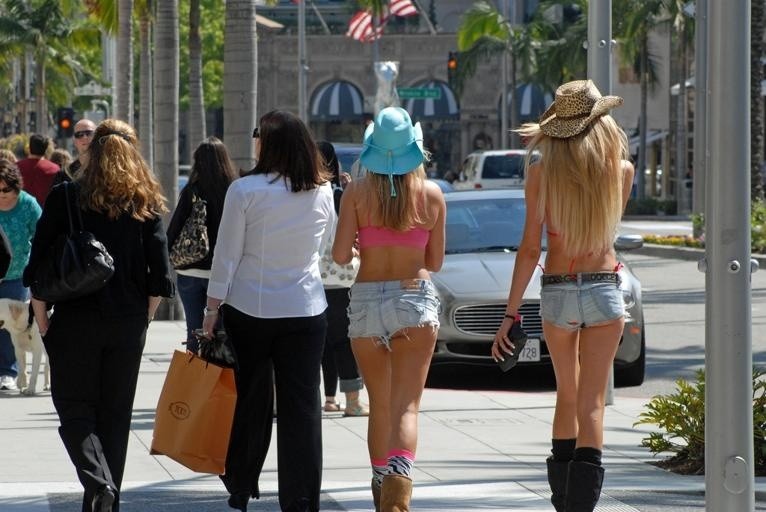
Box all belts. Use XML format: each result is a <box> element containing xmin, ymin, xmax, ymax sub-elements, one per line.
<box><xmin>543</xmin><ymin>272</ymin><xmax>618</xmax><ymax>286</ymax></box>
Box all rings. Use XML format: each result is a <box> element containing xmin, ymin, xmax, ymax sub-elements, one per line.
<box><xmin>493</xmin><ymin>343</ymin><xmax>499</xmax><ymax>346</ymax></box>
<box><xmin>203</xmin><ymin>330</ymin><xmax>208</xmax><ymax>335</ymax></box>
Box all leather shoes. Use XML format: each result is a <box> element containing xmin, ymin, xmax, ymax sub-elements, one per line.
<box><xmin>92</xmin><ymin>485</ymin><xmax>117</xmax><ymax>511</ymax></box>
<box><xmin>228</xmin><ymin>494</ymin><xmax>249</xmax><ymax>511</ymax></box>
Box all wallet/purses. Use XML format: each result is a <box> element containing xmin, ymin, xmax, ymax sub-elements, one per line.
<box><xmin>496</xmin><ymin>320</ymin><xmax>527</xmax><ymax>371</ymax></box>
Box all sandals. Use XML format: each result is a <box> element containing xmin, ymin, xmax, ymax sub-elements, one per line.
<box><xmin>342</xmin><ymin>398</ymin><xmax>370</xmax><ymax>416</ymax></box>
<box><xmin>324</xmin><ymin>401</ymin><xmax>346</xmax><ymax>411</ymax></box>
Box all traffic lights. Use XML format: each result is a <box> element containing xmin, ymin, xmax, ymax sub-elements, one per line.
<box><xmin>58</xmin><ymin>108</ymin><xmax>73</xmax><ymax>139</ymax></box>
<box><xmin>449</xmin><ymin>53</ymin><xmax>459</xmax><ymax>79</ymax></box>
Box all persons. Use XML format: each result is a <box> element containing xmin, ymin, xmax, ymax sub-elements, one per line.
<box><xmin>420</xmin><ymin>120</ymin><xmax>493</xmax><ymax>185</ymax></box>
<box><xmin>1</xmin><ymin>118</ymin><xmax>99</xmax><ymax>398</ymax></box>
<box><xmin>330</xmin><ymin>106</ymin><xmax>447</xmax><ymax>511</ymax></box>
<box><xmin>311</xmin><ymin>141</ymin><xmax>372</xmax><ymax>417</ymax></box>
<box><xmin>164</xmin><ymin>133</ymin><xmax>240</xmax><ymax>354</ymax></box>
<box><xmin>21</xmin><ymin>120</ymin><xmax>176</xmax><ymax>512</ymax></box>
<box><xmin>201</xmin><ymin>108</ymin><xmax>337</xmax><ymax>512</ymax></box>
<box><xmin>490</xmin><ymin>78</ymin><xmax>636</xmax><ymax>512</ymax></box>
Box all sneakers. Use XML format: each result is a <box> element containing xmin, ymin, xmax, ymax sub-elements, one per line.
<box><xmin>0</xmin><ymin>375</ymin><xmax>18</xmax><ymax>390</ymax></box>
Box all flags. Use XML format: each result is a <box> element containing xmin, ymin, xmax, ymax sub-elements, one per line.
<box><xmin>343</xmin><ymin>0</ymin><xmax>419</xmax><ymax>46</ymax></box>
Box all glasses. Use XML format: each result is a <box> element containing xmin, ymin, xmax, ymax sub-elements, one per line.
<box><xmin>0</xmin><ymin>186</ymin><xmax>14</xmax><ymax>193</ymax></box>
<box><xmin>73</xmin><ymin>130</ymin><xmax>93</xmax><ymax>138</ymax></box>
<box><xmin>252</xmin><ymin>128</ymin><xmax>262</xmax><ymax>139</ymax></box>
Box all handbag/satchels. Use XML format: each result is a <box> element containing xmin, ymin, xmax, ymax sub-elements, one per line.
<box><xmin>33</xmin><ymin>230</ymin><xmax>115</xmax><ymax>303</ymax></box>
<box><xmin>320</xmin><ymin>255</ymin><xmax>361</xmax><ymax>289</ymax></box>
<box><xmin>150</xmin><ymin>350</ymin><xmax>237</xmax><ymax>476</ymax></box>
<box><xmin>169</xmin><ymin>201</ymin><xmax>209</xmax><ymax>268</ymax></box>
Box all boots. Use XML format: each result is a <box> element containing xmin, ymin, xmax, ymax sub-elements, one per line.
<box><xmin>380</xmin><ymin>474</ymin><xmax>412</xmax><ymax>512</ymax></box>
<box><xmin>568</xmin><ymin>462</ymin><xmax>605</xmax><ymax>511</ymax></box>
<box><xmin>371</xmin><ymin>478</ymin><xmax>381</xmax><ymax>511</ymax></box>
<box><xmin>546</xmin><ymin>456</ymin><xmax>571</xmax><ymax>511</ymax></box>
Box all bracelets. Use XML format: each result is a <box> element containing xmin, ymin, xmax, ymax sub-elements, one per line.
<box><xmin>203</xmin><ymin>307</ymin><xmax>219</xmax><ymax>318</ymax></box>
<box><xmin>504</xmin><ymin>314</ymin><xmax>518</xmax><ymax>321</ymax></box>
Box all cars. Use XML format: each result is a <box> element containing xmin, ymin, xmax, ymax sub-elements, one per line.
<box><xmin>430</xmin><ymin>189</ymin><xmax>646</xmax><ymax>388</ymax></box>
<box><xmin>329</xmin><ymin>143</ymin><xmax>365</xmax><ymax>181</ymax></box>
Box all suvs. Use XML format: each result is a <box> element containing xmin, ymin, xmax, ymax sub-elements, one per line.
<box><xmin>458</xmin><ymin>150</ymin><xmax>544</xmax><ymax>187</ymax></box>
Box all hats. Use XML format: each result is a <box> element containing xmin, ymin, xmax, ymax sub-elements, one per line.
<box><xmin>359</xmin><ymin>107</ymin><xmax>424</xmax><ymax>197</ymax></box>
<box><xmin>539</xmin><ymin>80</ymin><xmax>624</xmax><ymax>139</ymax></box>
